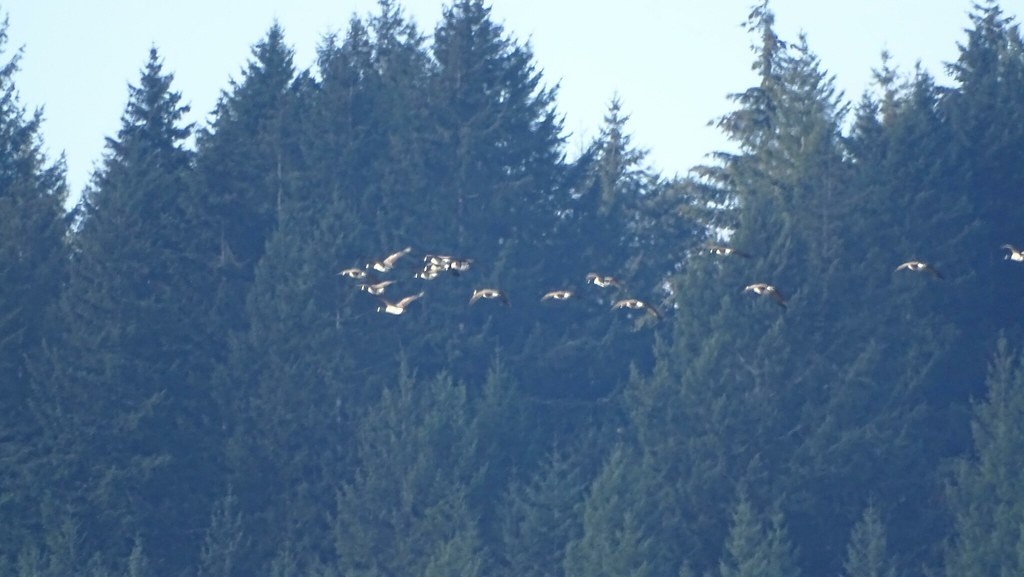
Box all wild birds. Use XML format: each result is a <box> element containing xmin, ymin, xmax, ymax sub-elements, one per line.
<box><xmin>736</xmin><ymin>280</ymin><xmax>788</xmax><ymax>311</ymax></box>
<box><xmin>584</xmin><ymin>271</ymin><xmax>625</xmax><ymax>289</ymax></box>
<box><xmin>468</xmin><ymin>287</ymin><xmax>511</xmax><ymax>308</ymax></box>
<box><xmin>695</xmin><ymin>244</ymin><xmax>750</xmax><ymax>259</ymax></box>
<box><xmin>607</xmin><ymin>296</ymin><xmax>660</xmax><ymax>319</ymax></box>
<box><xmin>893</xmin><ymin>257</ymin><xmax>945</xmax><ymax>281</ymax></box>
<box><xmin>539</xmin><ymin>282</ymin><xmax>582</xmax><ymax>304</ymax></box>
<box><xmin>999</xmin><ymin>241</ymin><xmax>1024</xmax><ymax>262</ymax></box>
<box><xmin>336</xmin><ymin>246</ymin><xmax>472</xmax><ymax>316</ymax></box>
